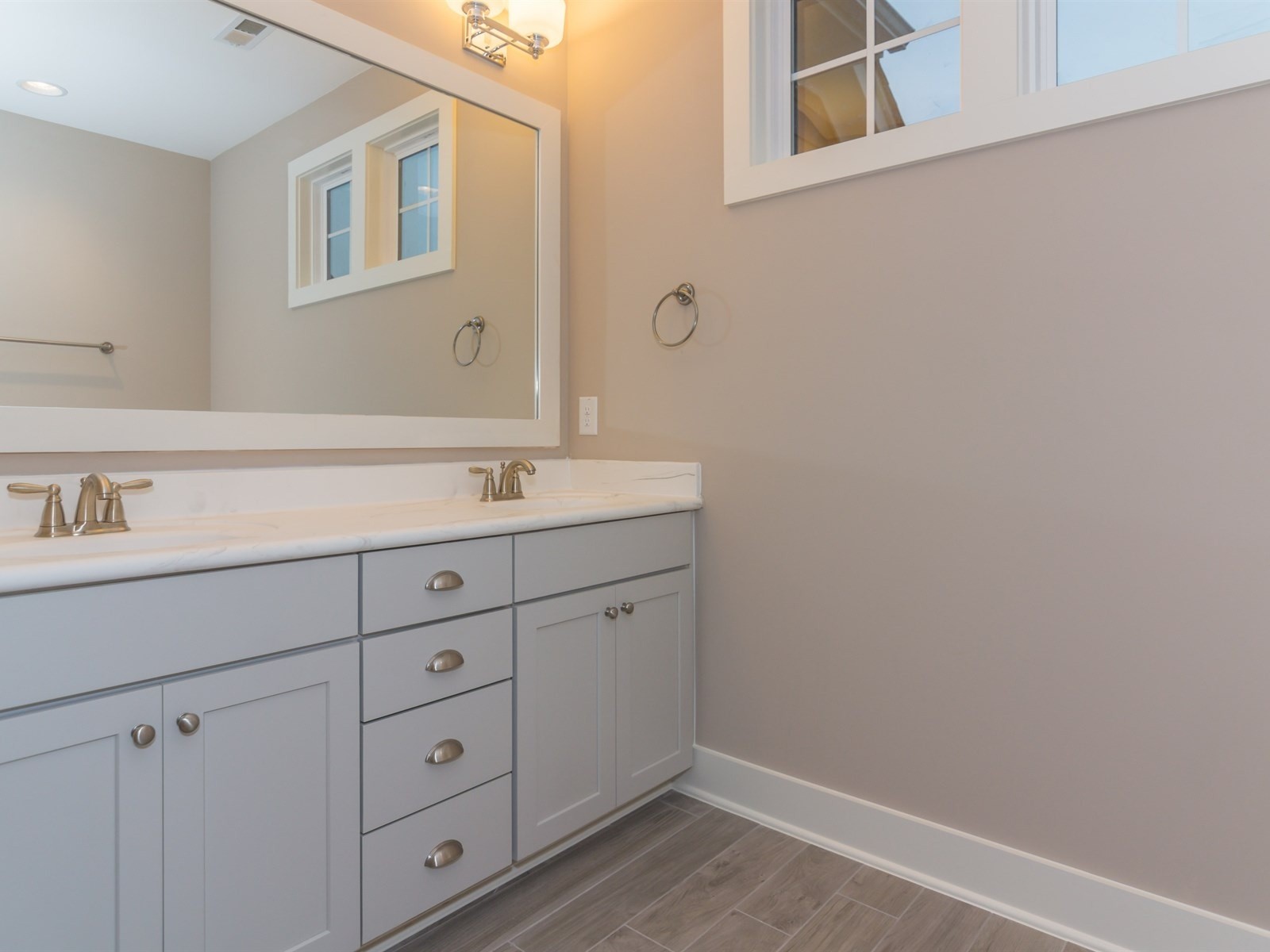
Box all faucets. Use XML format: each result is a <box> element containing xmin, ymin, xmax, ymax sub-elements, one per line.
<box><xmin>7</xmin><ymin>472</ymin><xmax>154</xmax><ymax>539</ymax></box>
<box><xmin>469</xmin><ymin>457</ymin><xmax>536</xmax><ymax>502</ymax></box>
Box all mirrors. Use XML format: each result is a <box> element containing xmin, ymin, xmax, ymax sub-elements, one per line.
<box><xmin>0</xmin><ymin>0</ymin><xmax>562</xmax><ymax>453</ymax></box>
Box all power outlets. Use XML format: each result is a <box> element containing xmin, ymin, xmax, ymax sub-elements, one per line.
<box><xmin>578</xmin><ymin>396</ymin><xmax>598</xmax><ymax>435</ymax></box>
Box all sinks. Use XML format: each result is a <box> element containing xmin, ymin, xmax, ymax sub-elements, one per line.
<box><xmin>482</xmin><ymin>494</ymin><xmax>617</xmax><ymax>509</ymax></box>
<box><xmin>0</xmin><ymin>528</ymin><xmax>219</xmax><ymax>556</ymax></box>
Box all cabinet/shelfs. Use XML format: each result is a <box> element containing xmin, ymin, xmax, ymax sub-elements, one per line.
<box><xmin>0</xmin><ymin>511</ymin><xmax>699</xmax><ymax>952</ymax></box>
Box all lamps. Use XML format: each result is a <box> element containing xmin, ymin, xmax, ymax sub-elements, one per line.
<box><xmin>444</xmin><ymin>0</ymin><xmax>569</xmax><ymax>65</ymax></box>
